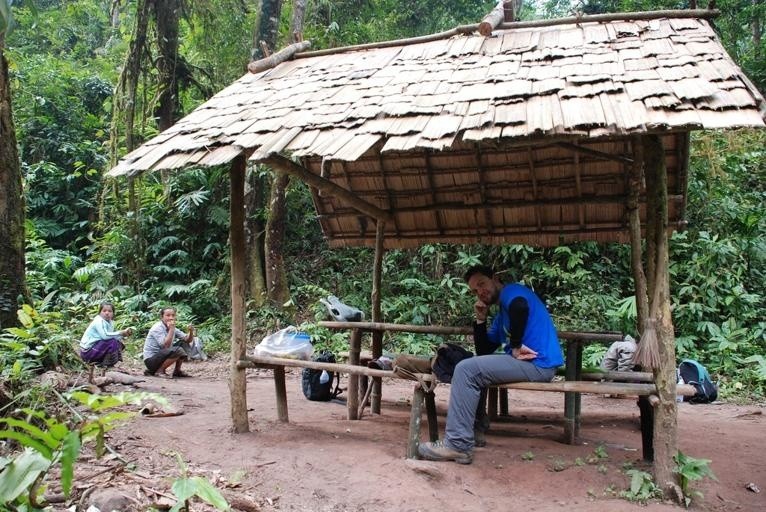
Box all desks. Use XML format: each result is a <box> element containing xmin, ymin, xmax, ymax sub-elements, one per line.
<box><xmin>318</xmin><ymin>320</ymin><xmax>624</xmax><ymax>446</ymax></box>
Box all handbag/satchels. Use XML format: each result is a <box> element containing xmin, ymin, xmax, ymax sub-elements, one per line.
<box><xmin>432</xmin><ymin>343</ymin><xmax>474</xmax><ymax>384</ymax></box>
<box><xmin>395</xmin><ymin>355</ymin><xmax>432</xmax><ymax>379</ymax></box>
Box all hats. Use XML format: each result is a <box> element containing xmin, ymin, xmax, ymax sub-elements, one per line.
<box><xmin>368</xmin><ymin>355</ymin><xmax>394</xmax><ymax>370</ymax></box>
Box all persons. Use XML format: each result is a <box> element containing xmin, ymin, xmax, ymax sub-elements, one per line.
<box><xmin>143</xmin><ymin>307</ymin><xmax>194</xmax><ymax>380</ymax></box>
<box><xmin>598</xmin><ymin>333</ymin><xmax>641</xmax><ymax>398</ymax></box>
<box><xmin>80</xmin><ymin>302</ymin><xmax>132</xmax><ymax>371</ymax></box>
<box><xmin>417</xmin><ymin>264</ymin><xmax>564</xmax><ymax>465</ymax></box>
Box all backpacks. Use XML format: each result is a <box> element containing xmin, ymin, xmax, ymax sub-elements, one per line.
<box><xmin>302</xmin><ymin>351</ymin><xmax>343</xmax><ymax>402</ymax></box>
<box><xmin>679</xmin><ymin>359</ymin><xmax>718</xmax><ymax>405</ymax></box>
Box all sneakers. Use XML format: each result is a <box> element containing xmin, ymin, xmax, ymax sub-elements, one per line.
<box><xmin>417</xmin><ymin>431</ymin><xmax>486</xmax><ymax>465</ymax></box>
<box><xmin>154</xmin><ymin>371</ymin><xmax>192</xmax><ymax>379</ymax></box>
